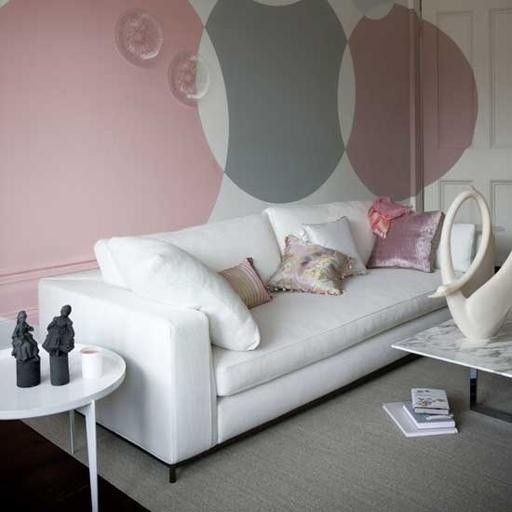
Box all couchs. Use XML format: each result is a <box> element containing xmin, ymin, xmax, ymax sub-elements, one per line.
<box><xmin>36</xmin><ymin>221</ymin><xmax>495</xmax><ymax>485</ymax></box>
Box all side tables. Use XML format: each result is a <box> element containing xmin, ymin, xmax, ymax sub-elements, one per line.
<box><xmin>0</xmin><ymin>343</ymin><xmax>127</xmax><ymax>512</ymax></box>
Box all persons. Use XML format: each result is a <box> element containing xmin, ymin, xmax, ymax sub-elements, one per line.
<box><xmin>11</xmin><ymin>311</ymin><xmax>41</xmax><ymax>361</ymax></box>
<box><xmin>43</xmin><ymin>304</ymin><xmax>76</xmax><ymax>360</ymax></box>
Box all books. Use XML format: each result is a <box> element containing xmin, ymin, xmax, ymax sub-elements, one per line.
<box><xmin>382</xmin><ymin>387</ymin><xmax>459</xmax><ymax>439</ymax></box>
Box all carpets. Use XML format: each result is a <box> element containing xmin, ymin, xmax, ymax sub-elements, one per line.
<box><xmin>24</xmin><ymin>354</ymin><xmax>512</xmax><ymax>512</ymax></box>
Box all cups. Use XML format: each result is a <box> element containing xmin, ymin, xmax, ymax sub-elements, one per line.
<box><xmin>80</xmin><ymin>345</ymin><xmax>103</xmax><ymax>379</ymax></box>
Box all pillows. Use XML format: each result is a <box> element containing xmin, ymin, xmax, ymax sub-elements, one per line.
<box><xmin>216</xmin><ymin>257</ymin><xmax>273</xmax><ymax>310</ymax></box>
<box><xmin>109</xmin><ymin>237</ymin><xmax>261</xmax><ymax>352</ymax></box>
<box><xmin>302</xmin><ymin>215</ymin><xmax>368</xmax><ymax>279</ymax></box>
<box><xmin>366</xmin><ymin>209</ymin><xmax>443</xmax><ymax>274</ymax></box>
<box><xmin>266</xmin><ymin>234</ymin><xmax>354</xmax><ymax>296</ymax></box>
